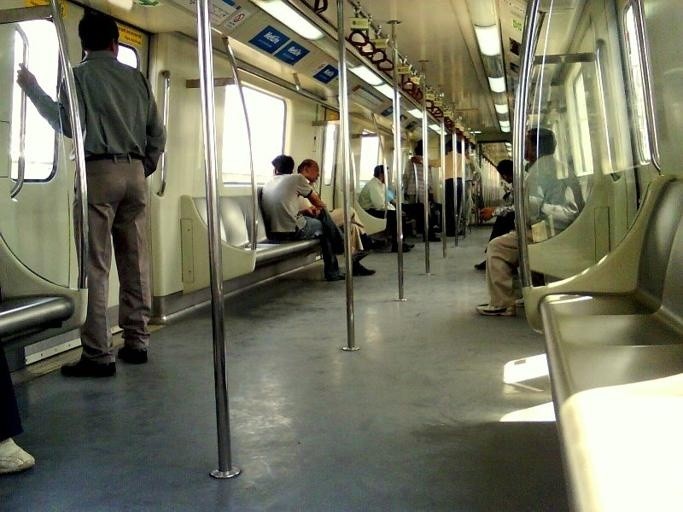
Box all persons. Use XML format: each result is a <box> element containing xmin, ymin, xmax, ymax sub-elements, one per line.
<box><xmin>403</xmin><ymin>135</ymin><xmax>530</xmax><ymax>269</ymax></box>
<box><xmin>261</xmin><ymin>154</ymin><xmax>346</xmax><ymax>281</ymax></box>
<box><xmin>17</xmin><ymin>8</ymin><xmax>164</xmax><ymax>376</ymax></box>
<box><xmin>297</xmin><ymin>159</ymin><xmax>377</xmax><ymax>276</ymax></box>
<box><xmin>384</xmin><ymin>184</ymin><xmax>414</xmax><ymax>248</ymax></box>
<box><xmin>1</xmin><ymin>344</ymin><xmax>36</xmax><ymax>473</ymax></box>
<box><xmin>476</xmin><ymin>127</ymin><xmax>588</xmax><ymax>316</ymax></box>
<box><xmin>360</xmin><ymin>164</ymin><xmax>415</xmax><ymax>252</ymax></box>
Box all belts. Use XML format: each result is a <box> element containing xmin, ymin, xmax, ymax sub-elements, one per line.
<box><xmin>86</xmin><ymin>151</ymin><xmax>141</xmax><ymax>161</ymax></box>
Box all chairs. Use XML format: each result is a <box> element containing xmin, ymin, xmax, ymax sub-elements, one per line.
<box><xmin>523</xmin><ymin>175</ymin><xmax>629</xmax><ymax>282</ymax></box>
<box><xmin>350</xmin><ymin>194</ymin><xmax>387</xmax><ymax>240</ymax></box>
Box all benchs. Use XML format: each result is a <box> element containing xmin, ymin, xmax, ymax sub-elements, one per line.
<box><xmin>179</xmin><ymin>191</ymin><xmax>320</xmax><ymax>291</ymax></box>
<box><xmin>537</xmin><ymin>180</ymin><xmax>681</xmax><ymax>505</ymax></box>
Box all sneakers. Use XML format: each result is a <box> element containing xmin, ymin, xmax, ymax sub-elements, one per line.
<box><xmin>474</xmin><ymin>259</ymin><xmax>486</xmax><ymax>270</ymax></box>
<box><xmin>117</xmin><ymin>346</ymin><xmax>148</xmax><ymax>364</ymax></box>
<box><xmin>61</xmin><ymin>353</ymin><xmax>116</xmax><ymax>378</ymax></box>
<box><xmin>475</xmin><ymin>296</ymin><xmax>524</xmax><ymax>317</ymax></box>
<box><xmin>327</xmin><ymin>273</ymin><xmax>345</xmax><ymax>280</ymax></box>
<box><xmin>0</xmin><ymin>436</ymin><xmax>35</xmax><ymax>474</ymax></box>
<box><xmin>352</xmin><ymin>252</ymin><xmax>368</xmax><ymax>263</ymax></box>
<box><xmin>353</xmin><ymin>265</ymin><xmax>375</xmax><ymax>276</ymax></box>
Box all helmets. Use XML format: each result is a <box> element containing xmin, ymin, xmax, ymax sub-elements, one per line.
<box><xmin>391</xmin><ymin>242</ymin><xmax>414</xmax><ymax>253</ymax></box>
